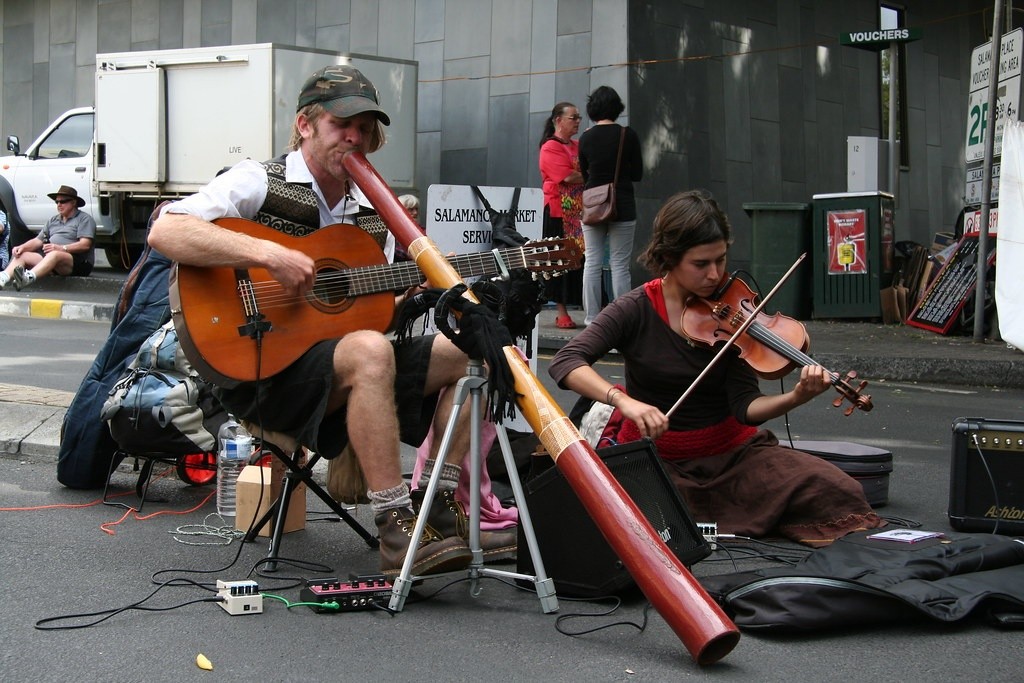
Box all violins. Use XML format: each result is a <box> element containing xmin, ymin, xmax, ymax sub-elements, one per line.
<box><xmin>680</xmin><ymin>272</ymin><xmax>874</xmax><ymax>418</ymax></box>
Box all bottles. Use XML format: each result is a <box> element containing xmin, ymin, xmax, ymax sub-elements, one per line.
<box><xmin>216</xmin><ymin>413</ymin><xmax>252</xmax><ymax>517</ymax></box>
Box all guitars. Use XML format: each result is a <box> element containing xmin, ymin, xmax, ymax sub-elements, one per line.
<box><xmin>167</xmin><ymin>216</ymin><xmax>587</xmax><ymax>390</ymax></box>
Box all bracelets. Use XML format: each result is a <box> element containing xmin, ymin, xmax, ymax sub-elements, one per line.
<box><xmin>607</xmin><ymin>385</ymin><xmax>624</xmax><ymax>403</ymax></box>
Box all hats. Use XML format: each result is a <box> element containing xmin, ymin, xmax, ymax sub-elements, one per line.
<box><xmin>295</xmin><ymin>64</ymin><xmax>391</xmax><ymax>127</ymax></box>
<box><xmin>47</xmin><ymin>185</ymin><xmax>86</xmax><ymax>207</ymax></box>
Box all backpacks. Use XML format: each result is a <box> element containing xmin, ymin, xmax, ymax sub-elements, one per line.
<box><xmin>470</xmin><ymin>185</ymin><xmax>551</xmax><ymax>347</ymax></box>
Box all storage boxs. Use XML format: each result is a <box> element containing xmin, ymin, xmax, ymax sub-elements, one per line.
<box><xmin>234</xmin><ymin>444</ymin><xmax>310</xmax><ymax>538</ymax></box>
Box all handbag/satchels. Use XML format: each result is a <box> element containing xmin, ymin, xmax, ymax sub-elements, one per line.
<box><xmin>582</xmin><ymin>182</ymin><xmax>618</xmax><ymax>226</ymax></box>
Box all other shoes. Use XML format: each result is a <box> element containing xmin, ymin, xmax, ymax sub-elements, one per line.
<box><xmin>13</xmin><ymin>265</ymin><xmax>34</xmax><ymax>292</ymax></box>
<box><xmin>556</xmin><ymin>315</ymin><xmax>576</xmax><ymax>328</ymax></box>
<box><xmin>0</xmin><ymin>276</ymin><xmax>7</xmax><ymax>290</ymax></box>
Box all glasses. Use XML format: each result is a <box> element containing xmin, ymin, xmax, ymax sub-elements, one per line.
<box><xmin>55</xmin><ymin>198</ymin><xmax>72</xmax><ymax>204</ymax></box>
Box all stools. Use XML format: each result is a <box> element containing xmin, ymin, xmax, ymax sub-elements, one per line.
<box><xmin>244</xmin><ymin>420</ymin><xmax>382</xmax><ymax>571</ymax></box>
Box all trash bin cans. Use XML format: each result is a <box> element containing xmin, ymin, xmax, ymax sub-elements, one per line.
<box><xmin>811</xmin><ymin>190</ymin><xmax>897</xmax><ymax>322</ymax></box>
<box><xmin>741</xmin><ymin>199</ymin><xmax>813</xmax><ymax>322</ymax></box>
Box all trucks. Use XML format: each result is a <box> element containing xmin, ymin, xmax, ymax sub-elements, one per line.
<box><xmin>0</xmin><ymin>43</ymin><xmax>417</xmax><ymax>268</ymax></box>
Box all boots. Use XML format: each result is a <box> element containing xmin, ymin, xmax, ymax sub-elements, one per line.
<box><xmin>409</xmin><ymin>490</ymin><xmax>517</xmax><ymax>564</ymax></box>
<box><xmin>374</xmin><ymin>503</ymin><xmax>474</xmax><ymax>589</ymax></box>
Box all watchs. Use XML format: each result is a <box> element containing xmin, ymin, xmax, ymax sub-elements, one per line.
<box><xmin>63</xmin><ymin>245</ymin><xmax>69</xmax><ymax>252</ymax></box>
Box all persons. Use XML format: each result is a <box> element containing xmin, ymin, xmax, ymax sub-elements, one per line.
<box><xmin>148</xmin><ymin>64</ymin><xmax>519</xmax><ymax>588</ymax></box>
<box><xmin>547</xmin><ymin>190</ymin><xmax>890</xmax><ymax>550</ymax></box>
<box><xmin>536</xmin><ymin>86</ymin><xmax>649</xmax><ymax>330</ymax></box>
<box><xmin>0</xmin><ymin>186</ymin><xmax>98</xmax><ymax>290</ymax></box>
